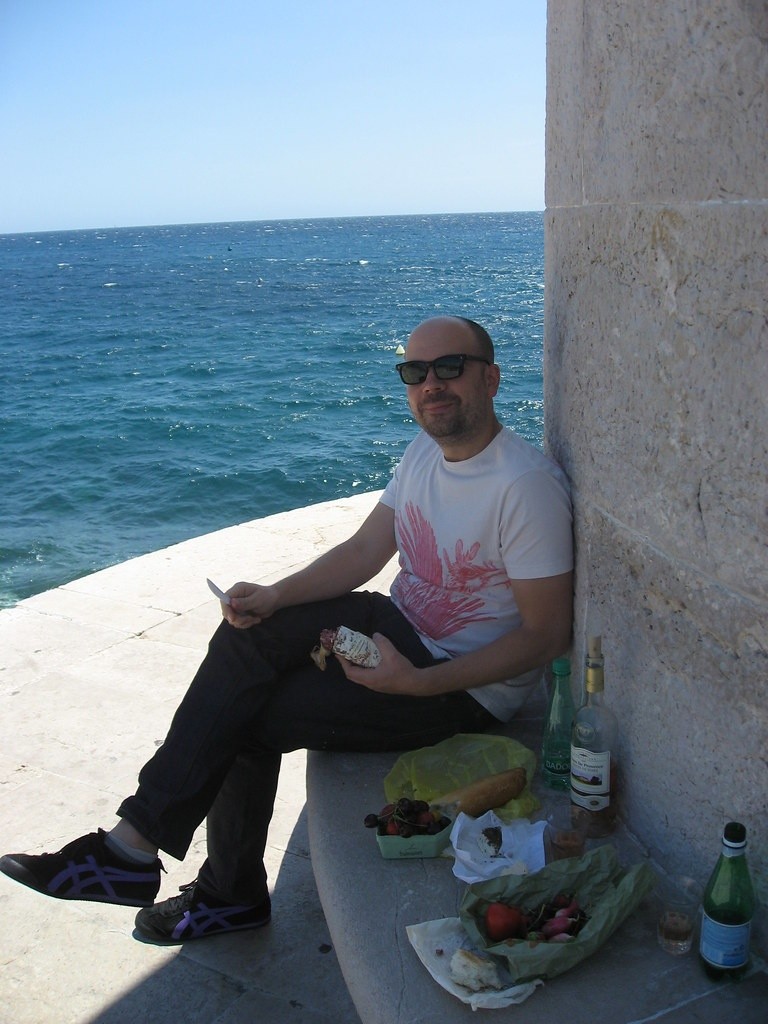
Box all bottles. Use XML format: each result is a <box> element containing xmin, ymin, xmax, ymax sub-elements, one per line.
<box><xmin>698</xmin><ymin>821</ymin><xmax>754</xmax><ymax>981</ymax></box>
<box><xmin>539</xmin><ymin>659</ymin><xmax>577</xmax><ymax>791</ymax></box>
<box><xmin>568</xmin><ymin>634</ymin><xmax>621</xmax><ymax>837</ymax></box>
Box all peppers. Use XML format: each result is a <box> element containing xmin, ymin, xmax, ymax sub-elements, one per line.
<box><xmin>484</xmin><ymin>891</ymin><xmax>523</xmax><ymax>942</ymax></box>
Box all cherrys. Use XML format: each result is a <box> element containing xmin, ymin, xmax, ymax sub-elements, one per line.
<box><xmin>363</xmin><ymin>798</ymin><xmax>451</xmax><ymax>839</ymax></box>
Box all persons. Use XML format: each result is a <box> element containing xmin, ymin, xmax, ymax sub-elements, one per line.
<box><xmin>0</xmin><ymin>315</ymin><xmax>576</xmax><ymax>946</ymax></box>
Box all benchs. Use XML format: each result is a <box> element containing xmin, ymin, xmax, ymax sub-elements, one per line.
<box><xmin>300</xmin><ymin>720</ymin><xmax>762</xmax><ymax>1024</ymax></box>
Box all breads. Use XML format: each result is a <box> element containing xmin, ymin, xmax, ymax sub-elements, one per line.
<box><xmin>318</xmin><ymin>625</ymin><xmax>382</xmax><ymax>669</ymax></box>
<box><xmin>428</xmin><ymin>766</ymin><xmax>527</xmax><ymax>817</ymax></box>
<box><xmin>450</xmin><ymin>949</ymin><xmax>501</xmax><ymax>991</ymax></box>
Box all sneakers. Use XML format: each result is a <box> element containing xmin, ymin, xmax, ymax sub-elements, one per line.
<box><xmin>0</xmin><ymin>827</ymin><xmax>162</xmax><ymax>908</ymax></box>
<box><xmin>131</xmin><ymin>877</ymin><xmax>272</xmax><ymax>946</ymax></box>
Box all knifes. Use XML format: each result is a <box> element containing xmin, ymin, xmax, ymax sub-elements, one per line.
<box><xmin>206</xmin><ymin>578</ymin><xmax>246</xmax><ymax>616</ymax></box>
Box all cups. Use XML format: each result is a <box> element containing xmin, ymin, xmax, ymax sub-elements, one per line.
<box><xmin>652</xmin><ymin>873</ymin><xmax>703</xmax><ymax>957</ymax></box>
<box><xmin>546</xmin><ymin>806</ymin><xmax>591</xmax><ymax>861</ymax></box>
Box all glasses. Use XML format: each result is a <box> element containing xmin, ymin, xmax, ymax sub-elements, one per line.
<box><xmin>395</xmin><ymin>354</ymin><xmax>490</xmax><ymax>386</ymax></box>
